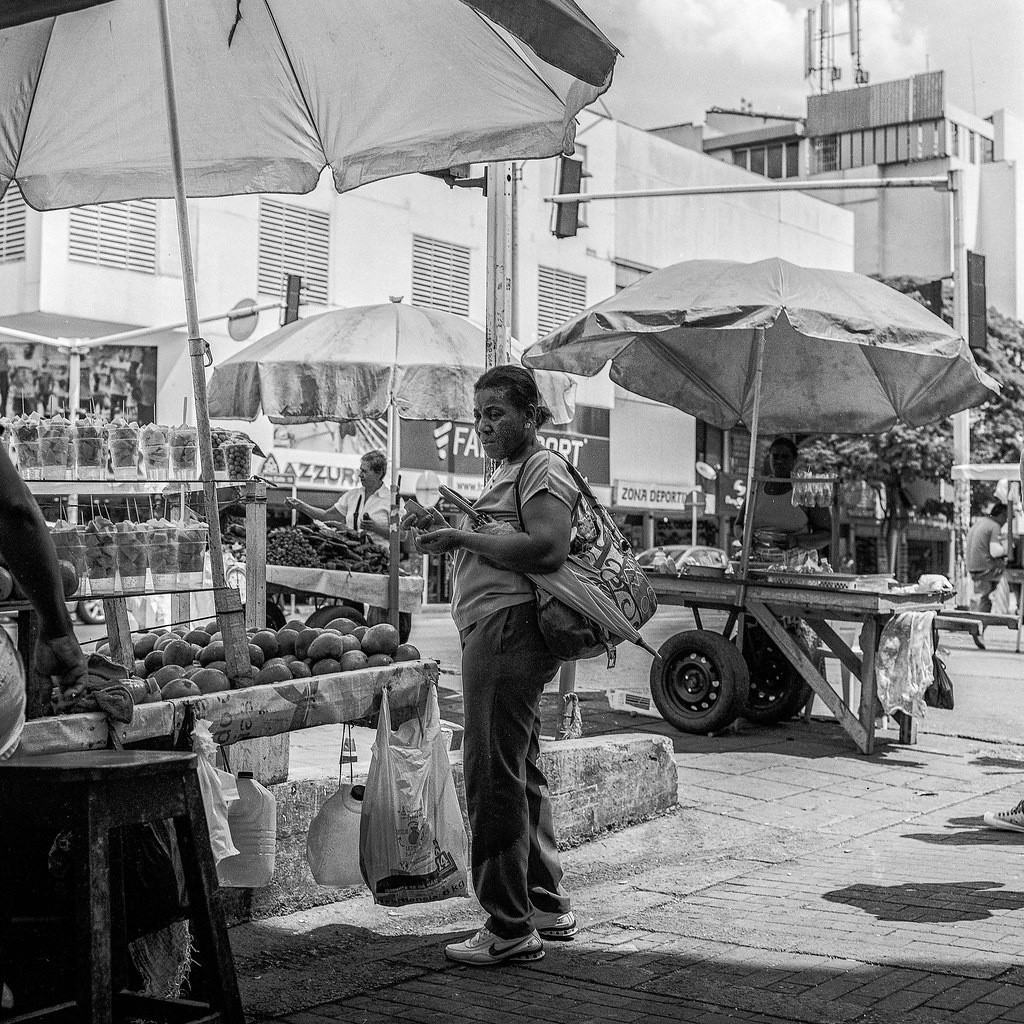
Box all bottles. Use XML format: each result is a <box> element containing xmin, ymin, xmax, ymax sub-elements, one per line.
<box><xmin>306</xmin><ymin>781</ymin><xmax>368</xmax><ymax>886</ymax></box>
<box><xmin>821</xmin><ymin>557</ymin><xmax>834</xmax><ymax>574</ymax></box>
<box><xmin>340</xmin><ymin>738</ymin><xmax>357</xmax><ymax>763</ymax></box>
<box><xmin>653</xmin><ymin>546</ymin><xmax>677</xmax><ymax>575</ymax></box>
<box><xmin>216</xmin><ymin>771</ymin><xmax>276</xmax><ymax>888</ymax></box>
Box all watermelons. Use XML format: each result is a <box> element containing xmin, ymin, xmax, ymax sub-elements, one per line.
<box><xmin>49</xmin><ymin>517</ymin><xmax>209</xmax><ymax>578</ymax></box>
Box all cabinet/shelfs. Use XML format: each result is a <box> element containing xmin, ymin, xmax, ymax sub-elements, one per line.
<box><xmin>0</xmin><ymin>478</ymin><xmax>267</xmax><ymax>678</ymax></box>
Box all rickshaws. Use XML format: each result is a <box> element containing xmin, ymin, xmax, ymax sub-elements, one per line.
<box><xmin>637</xmin><ymin>565</ymin><xmax>1019</xmax><ymax>750</ymax></box>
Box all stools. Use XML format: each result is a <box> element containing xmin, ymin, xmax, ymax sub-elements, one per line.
<box><xmin>0</xmin><ymin>750</ymin><xmax>246</xmax><ymax>1024</ymax></box>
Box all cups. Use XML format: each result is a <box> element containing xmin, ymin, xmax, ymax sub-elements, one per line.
<box><xmin>0</xmin><ymin>420</ymin><xmax>254</xmax><ymax>487</ymax></box>
<box><xmin>51</xmin><ymin>532</ymin><xmax>88</xmax><ymax>596</ymax></box>
<box><xmin>148</xmin><ymin>528</ymin><xmax>179</xmax><ymax>590</ymax></box>
<box><xmin>176</xmin><ymin>529</ymin><xmax>207</xmax><ymax>586</ymax></box>
<box><xmin>80</xmin><ymin>532</ymin><xmax>117</xmax><ymax>594</ymax></box>
<box><xmin>116</xmin><ymin>531</ymin><xmax>148</xmax><ymax>592</ymax></box>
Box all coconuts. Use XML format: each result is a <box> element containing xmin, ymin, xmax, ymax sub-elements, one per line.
<box><xmin>88</xmin><ymin>619</ymin><xmax>420</xmax><ymax>702</ymax></box>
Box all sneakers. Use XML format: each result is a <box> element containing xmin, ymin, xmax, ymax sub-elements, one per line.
<box><xmin>531</xmin><ymin>905</ymin><xmax>579</xmax><ymax>937</ymax></box>
<box><xmin>984</xmin><ymin>798</ymin><xmax>1024</xmax><ymax>832</ymax></box>
<box><xmin>443</xmin><ymin>926</ymin><xmax>545</xmax><ymax>965</ymax></box>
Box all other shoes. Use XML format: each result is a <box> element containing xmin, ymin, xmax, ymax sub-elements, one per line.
<box><xmin>974</xmin><ymin>635</ymin><xmax>987</xmax><ymax>650</ymax></box>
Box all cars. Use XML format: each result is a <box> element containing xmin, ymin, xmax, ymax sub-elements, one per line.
<box><xmin>631</xmin><ymin>544</ymin><xmax>730</xmax><ymax>574</ymax></box>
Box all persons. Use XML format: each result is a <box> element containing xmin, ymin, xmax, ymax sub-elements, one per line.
<box><xmin>966</xmin><ymin>503</ymin><xmax>1008</xmax><ymax>650</ymax></box>
<box><xmin>285</xmin><ymin>451</ymin><xmax>409</xmax><ymax>551</ymax></box>
<box><xmin>734</xmin><ymin>437</ymin><xmax>833</xmax><ymax>720</ymax></box>
<box><xmin>399</xmin><ymin>365</ymin><xmax>583</xmax><ymax>966</ymax></box>
<box><xmin>0</xmin><ymin>424</ymin><xmax>88</xmax><ymax>708</ymax></box>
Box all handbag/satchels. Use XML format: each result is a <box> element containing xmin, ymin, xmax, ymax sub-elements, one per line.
<box><xmin>924</xmin><ymin>626</ymin><xmax>954</xmax><ymax>710</ymax></box>
<box><xmin>515</xmin><ymin>449</ymin><xmax>657</xmax><ymax>668</ymax></box>
<box><xmin>360</xmin><ymin>680</ymin><xmax>472</xmax><ymax>907</ymax></box>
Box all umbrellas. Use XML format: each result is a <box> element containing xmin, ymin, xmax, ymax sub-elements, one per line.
<box><xmin>211</xmin><ymin>297</ymin><xmax>578</xmax><ymax>627</ymax></box>
<box><xmin>439</xmin><ymin>485</ymin><xmax>664</xmax><ymax>662</ymax></box>
<box><xmin>0</xmin><ymin>0</ymin><xmax>624</xmax><ymax>588</ymax></box>
<box><xmin>521</xmin><ymin>257</ymin><xmax>1002</xmax><ymax>608</ymax></box>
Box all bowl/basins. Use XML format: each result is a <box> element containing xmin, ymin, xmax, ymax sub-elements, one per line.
<box><xmin>689</xmin><ymin>564</ymin><xmax>726</xmax><ymax>579</ymax></box>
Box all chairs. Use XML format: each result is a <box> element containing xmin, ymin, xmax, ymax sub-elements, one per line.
<box><xmin>803</xmin><ymin>579</ymin><xmax>887</xmax><ymax>729</ymax></box>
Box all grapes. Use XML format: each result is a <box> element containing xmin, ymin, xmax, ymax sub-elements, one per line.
<box><xmin>259</xmin><ymin>527</ymin><xmax>327</xmax><ymax>570</ymax></box>
<box><xmin>209</xmin><ymin>427</ymin><xmax>251</xmax><ymax>480</ymax></box>
